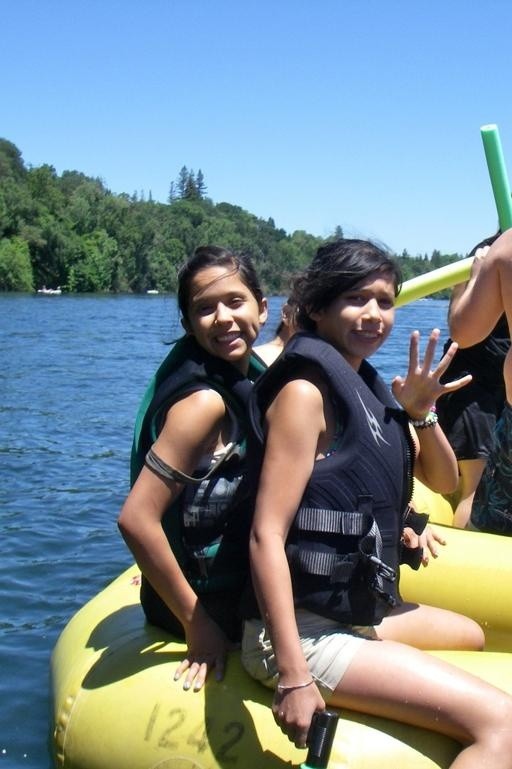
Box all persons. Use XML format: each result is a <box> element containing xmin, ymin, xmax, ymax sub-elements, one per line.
<box><xmin>429</xmin><ymin>229</ymin><xmax>509</xmax><ymax>498</ymax></box>
<box><xmin>252</xmin><ymin>278</ymin><xmax>302</xmax><ymax>368</ymax></box>
<box><xmin>115</xmin><ymin>244</ymin><xmax>268</xmax><ymax>694</ymax></box>
<box><xmin>227</xmin><ymin>237</ymin><xmax>511</xmax><ymax>769</ymax></box>
<box><xmin>452</xmin><ymin>219</ymin><xmax>512</xmax><ymax>537</ymax></box>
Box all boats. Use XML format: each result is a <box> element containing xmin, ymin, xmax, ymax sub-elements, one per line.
<box><xmin>50</xmin><ymin>459</ymin><xmax>512</xmax><ymax>769</ymax></box>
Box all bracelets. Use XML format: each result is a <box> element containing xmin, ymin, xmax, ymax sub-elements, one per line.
<box><xmin>275</xmin><ymin>679</ymin><xmax>315</xmax><ymax>691</ymax></box>
<box><xmin>408</xmin><ymin>407</ymin><xmax>440</xmax><ymax>430</ymax></box>
